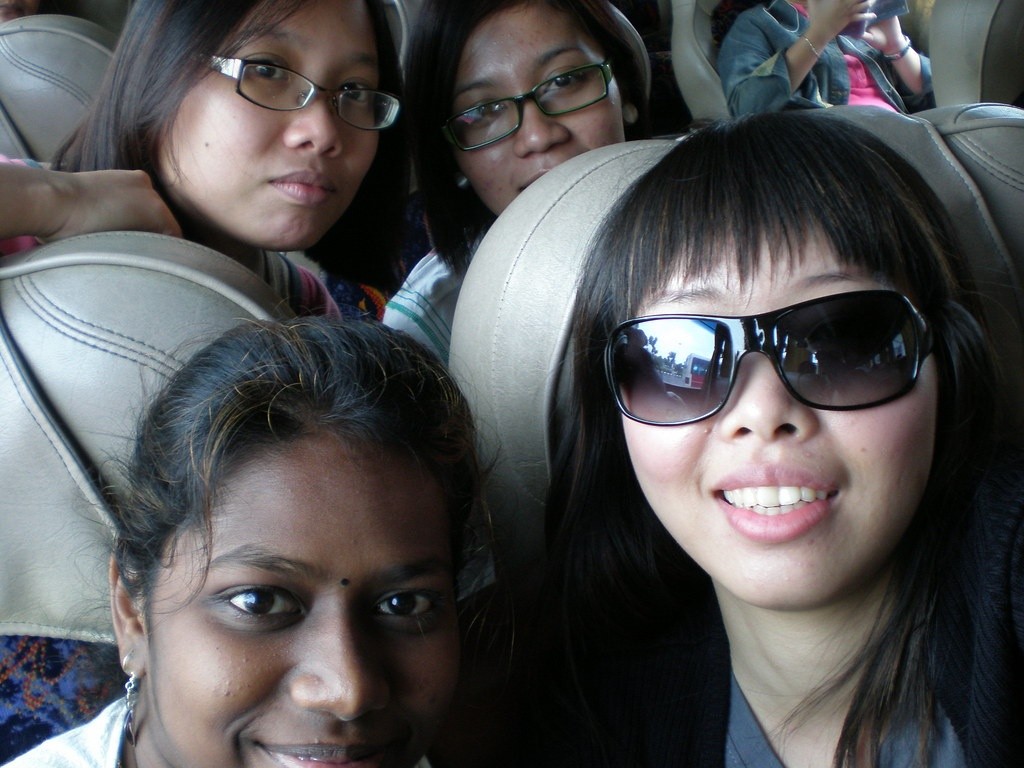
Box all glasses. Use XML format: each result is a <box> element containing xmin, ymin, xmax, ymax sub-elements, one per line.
<box><xmin>440</xmin><ymin>58</ymin><xmax>614</xmax><ymax>151</ymax></box>
<box><xmin>188</xmin><ymin>52</ymin><xmax>401</xmax><ymax>130</ymax></box>
<box><xmin>603</xmin><ymin>289</ymin><xmax>936</xmax><ymax>426</ymax></box>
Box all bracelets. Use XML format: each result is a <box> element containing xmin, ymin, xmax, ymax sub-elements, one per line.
<box><xmin>801</xmin><ymin>34</ymin><xmax>821</xmax><ymax>59</ymax></box>
<box><xmin>883</xmin><ymin>35</ymin><xmax>910</xmax><ymax>59</ymax></box>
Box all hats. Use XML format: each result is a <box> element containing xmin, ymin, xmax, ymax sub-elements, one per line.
<box><xmin>394</xmin><ymin>0</ymin><xmax>651</xmax><ymax>194</ymax></box>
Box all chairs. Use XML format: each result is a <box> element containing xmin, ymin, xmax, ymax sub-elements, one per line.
<box><xmin>0</xmin><ymin>0</ymin><xmax>1024</xmax><ymax>768</ymax></box>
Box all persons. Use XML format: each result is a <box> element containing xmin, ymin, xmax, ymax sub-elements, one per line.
<box><xmin>0</xmin><ymin>320</ymin><xmax>493</xmax><ymax>768</ymax></box>
<box><xmin>719</xmin><ymin>0</ymin><xmax>935</xmax><ymax>118</ymax></box>
<box><xmin>1</xmin><ymin>0</ymin><xmax>402</xmax><ymax>325</ymax></box>
<box><xmin>515</xmin><ymin>111</ymin><xmax>1024</xmax><ymax>768</ymax></box>
<box><xmin>382</xmin><ymin>0</ymin><xmax>645</xmax><ymax>365</ymax></box>
